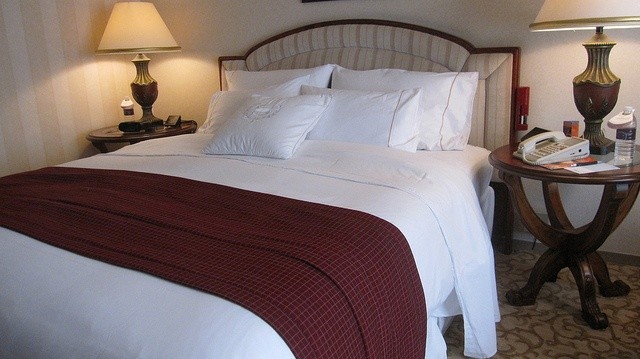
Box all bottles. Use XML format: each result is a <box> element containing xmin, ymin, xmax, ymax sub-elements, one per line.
<box><xmin>614</xmin><ymin>106</ymin><xmax>637</xmax><ymax>164</ymax></box>
<box><xmin>121</xmin><ymin>96</ymin><xmax>134</xmax><ymax>121</ymax></box>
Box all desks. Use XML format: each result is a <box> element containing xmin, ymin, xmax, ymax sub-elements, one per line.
<box><xmin>86</xmin><ymin>120</ymin><xmax>198</xmax><ymax>153</ymax></box>
<box><xmin>486</xmin><ymin>143</ymin><xmax>640</xmax><ymax>330</ymax></box>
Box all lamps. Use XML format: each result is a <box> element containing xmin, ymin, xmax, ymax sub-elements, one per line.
<box><xmin>528</xmin><ymin>1</ymin><xmax>640</xmax><ymax>164</ymax></box>
<box><xmin>94</xmin><ymin>1</ymin><xmax>182</xmax><ymax>129</ymax></box>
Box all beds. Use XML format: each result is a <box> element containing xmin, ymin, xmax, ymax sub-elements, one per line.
<box><xmin>1</xmin><ymin>18</ymin><xmax>522</xmax><ymax>359</ymax></box>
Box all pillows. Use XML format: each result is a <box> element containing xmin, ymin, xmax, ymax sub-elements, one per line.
<box><xmin>329</xmin><ymin>65</ymin><xmax>479</xmax><ymax>154</ymax></box>
<box><xmin>201</xmin><ymin>89</ymin><xmax>334</xmax><ymax>161</ymax></box>
<box><xmin>299</xmin><ymin>83</ymin><xmax>424</xmax><ymax>154</ymax></box>
<box><xmin>224</xmin><ymin>61</ymin><xmax>334</xmax><ymax>98</ymax></box>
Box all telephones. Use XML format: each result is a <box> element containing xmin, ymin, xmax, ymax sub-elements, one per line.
<box><xmin>513</xmin><ymin>131</ymin><xmax>590</xmax><ymax>166</ymax></box>
